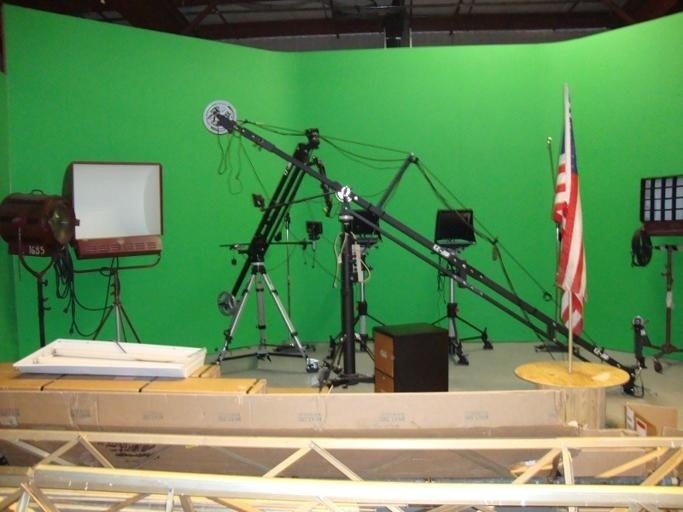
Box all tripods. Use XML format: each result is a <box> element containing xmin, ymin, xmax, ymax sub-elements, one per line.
<box><xmin>92</xmin><ymin>302</ymin><xmax>141</xmax><ymax>343</ymax></box>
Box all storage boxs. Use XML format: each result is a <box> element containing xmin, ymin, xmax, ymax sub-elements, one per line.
<box><xmin>625</xmin><ymin>401</ymin><xmax>683</xmax><ymax>479</ymax></box>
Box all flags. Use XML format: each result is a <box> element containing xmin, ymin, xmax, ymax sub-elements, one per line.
<box><xmin>552</xmin><ymin>101</ymin><xmax>585</xmax><ymax>335</ymax></box>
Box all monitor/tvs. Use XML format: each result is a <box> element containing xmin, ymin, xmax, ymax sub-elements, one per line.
<box><xmin>351</xmin><ymin>209</ymin><xmax>379</xmax><ymax>238</ymax></box>
<box><xmin>434</xmin><ymin>209</ymin><xmax>476</xmax><ymax>247</ymax></box>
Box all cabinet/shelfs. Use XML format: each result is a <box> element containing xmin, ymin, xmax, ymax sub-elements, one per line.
<box><xmin>372</xmin><ymin>327</ymin><xmax>447</xmax><ymax>392</ymax></box>
